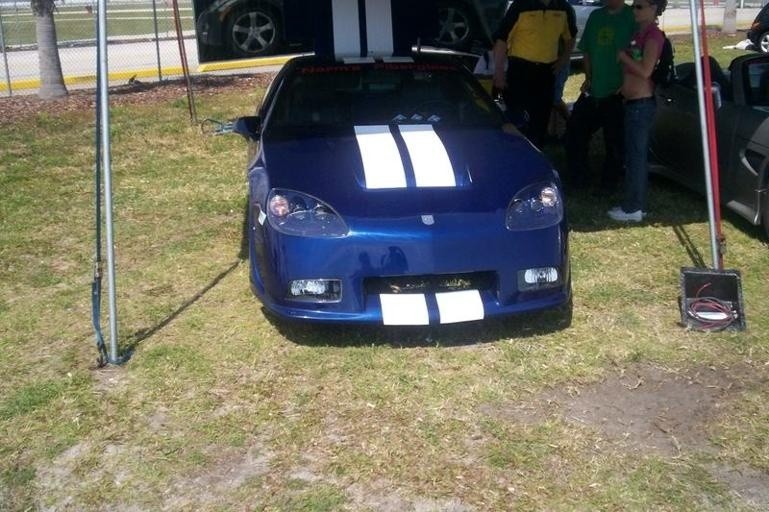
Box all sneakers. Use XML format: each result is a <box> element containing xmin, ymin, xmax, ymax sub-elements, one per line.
<box><xmin>565</xmin><ymin>181</ymin><xmax>647</xmax><ymax>223</ymax></box>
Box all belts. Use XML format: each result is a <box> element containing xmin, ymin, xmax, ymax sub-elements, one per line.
<box><xmin>622</xmin><ymin>95</ymin><xmax>658</xmax><ymax>106</ymax></box>
<box><xmin>508</xmin><ymin>56</ymin><xmax>556</xmax><ymax>67</ymax></box>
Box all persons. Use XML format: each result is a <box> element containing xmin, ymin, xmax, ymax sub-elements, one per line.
<box><xmin>560</xmin><ymin>1</ymin><xmax>638</xmax><ymax>196</ymax></box>
<box><xmin>490</xmin><ymin>0</ymin><xmax>579</xmax><ymax>149</ymax></box>
<box><xmin>474</xmin><ymin>31</ymin><xmax>508</xmax><ymax>76</ymax></box>
<box><xmin>546</xmin><ymin>33</ymin><xmax>577</xmax><ymax>145</ymax></box>
<box><xmin>605</xmin><ymin>0</ymin><xmax>667</xmax><ymax>223</ymax></box>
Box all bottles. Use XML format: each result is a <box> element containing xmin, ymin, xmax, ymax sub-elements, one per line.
<box><xmin>630</xmin><ymin>39</ymin><xmax>643</xmax><ymax>62</ymax></box>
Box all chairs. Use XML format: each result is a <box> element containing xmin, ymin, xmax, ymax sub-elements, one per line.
<box><xmin>690</xmin><ymin>55</ymin><xmax>732</xmax><ymax>102</ymax></box>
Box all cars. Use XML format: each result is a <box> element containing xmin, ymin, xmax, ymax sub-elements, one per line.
<box><xmin>233</xmin><ymin>58</ymin><xmax>571</xmax><ymax>327</ymax></box>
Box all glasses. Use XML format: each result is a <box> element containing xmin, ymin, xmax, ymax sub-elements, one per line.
<box><xmin>631</xmin><ymin>3</ymin><xmax>654</xmax><ymax>10</ymax></box>
